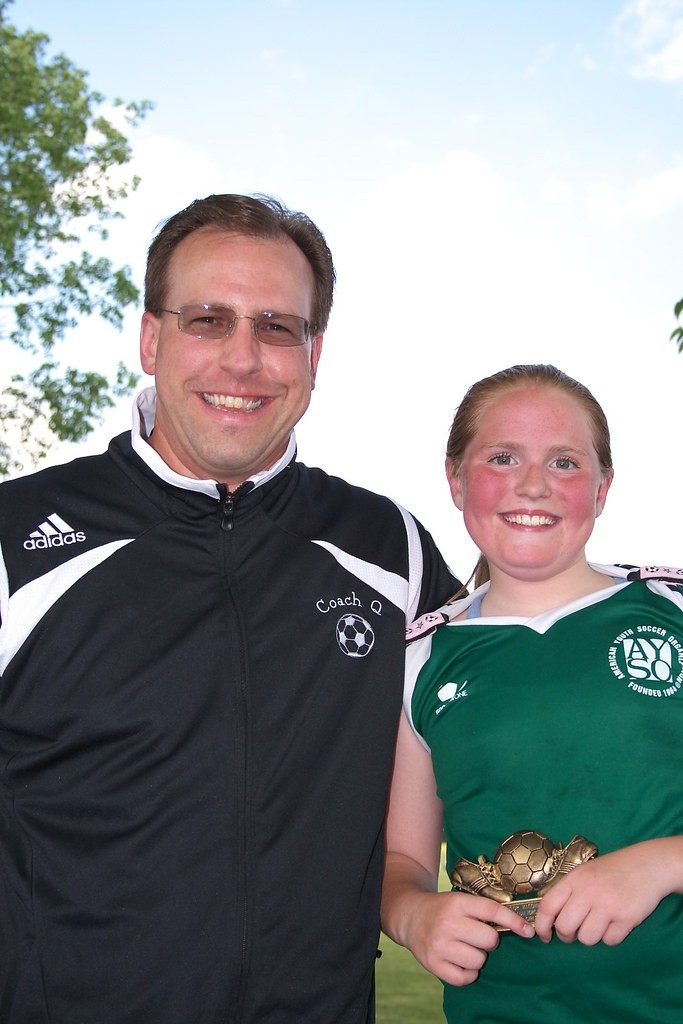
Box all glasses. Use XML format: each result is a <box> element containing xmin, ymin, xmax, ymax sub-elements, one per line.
<box><xmin>158</xmin><ymin>304</ymin><xmax>315</xmax><ymax>347</ymax></box>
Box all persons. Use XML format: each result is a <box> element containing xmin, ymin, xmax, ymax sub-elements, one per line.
<box><xmin>0</xmin><ymin>193</ymin><xmax>471</xmax><ymax>1024</ymax></box>
<box><xmin>380</xmin><ymin>366</ymin><xmax>683</xmax><ymax>1024</ymax></box>
